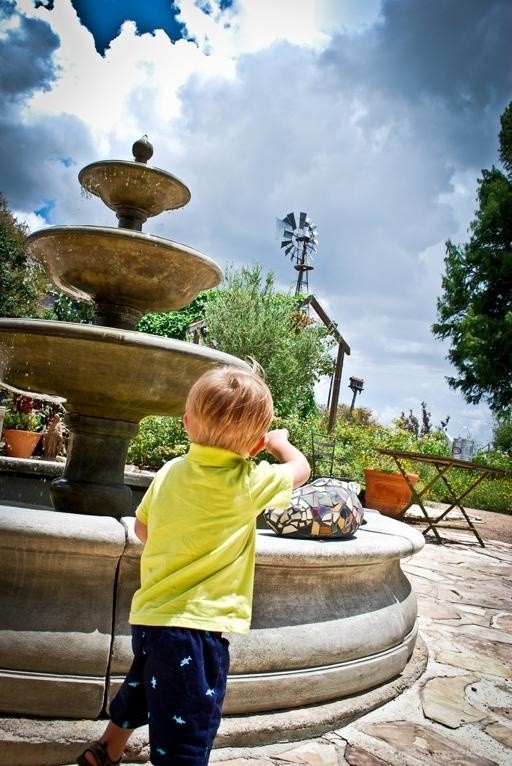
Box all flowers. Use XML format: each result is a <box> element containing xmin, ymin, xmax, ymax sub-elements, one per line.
<box><xmin>3</xmin><ymin>392</ymin><xmax>51</xmax><ymax>431</ymax></box>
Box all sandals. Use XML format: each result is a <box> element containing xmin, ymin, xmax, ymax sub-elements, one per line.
<box><xmin>75</xmin><ymin>740</ymin><xmax>123</xmax><ymax>766</ymax></box>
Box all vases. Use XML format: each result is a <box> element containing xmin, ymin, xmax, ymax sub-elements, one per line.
<box><xmin>4</xmin><ymin>427</ymin><xmax>42</xmax><ymax>457</ymax></box>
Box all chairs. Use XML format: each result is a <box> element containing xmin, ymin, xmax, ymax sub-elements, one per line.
<box><xmin>305</xmin><ymin>430</ymin><xmax>355</xmax><ymax>482</ymax></box>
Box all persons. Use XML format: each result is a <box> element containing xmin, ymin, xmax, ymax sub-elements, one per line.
<box><xmin>79</xmin><ymin>365</ymin><xmax>311</xmax><ymax>766</ymax></box>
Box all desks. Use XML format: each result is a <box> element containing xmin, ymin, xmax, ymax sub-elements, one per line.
<box><xmin>371</xmin><ymin>444</ymin><xmax>511</xmax><ymax>549</ymax></box>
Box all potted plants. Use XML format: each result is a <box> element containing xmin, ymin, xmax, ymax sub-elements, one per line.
<box><xmin>362</xmin><ymin>463</ymin><xmax>418</xmax><ymax>515</ymax></box>
